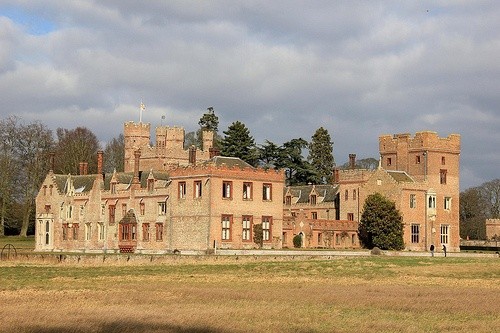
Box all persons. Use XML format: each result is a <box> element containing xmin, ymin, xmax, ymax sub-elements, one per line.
<box><xmin>430</xmin><ymin>244</ymin><xmax>435</xmax><ymax>257</ymax></box>
<box><xmin>442</xmin><ymin>244</ymin><xmax>446</xmax><ymax>257</ymax></box>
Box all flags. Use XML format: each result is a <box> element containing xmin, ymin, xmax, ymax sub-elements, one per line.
<box><xmin>140</xmin><ymin>102</ymin><xmax>146</xmax><ymax>111</ymax></box>
